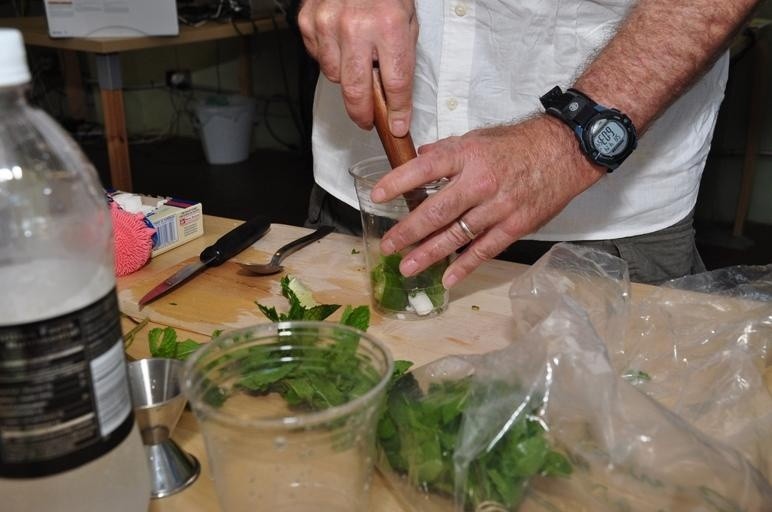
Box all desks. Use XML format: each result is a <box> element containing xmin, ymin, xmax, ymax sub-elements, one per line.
<box><xmin>2</xmin><ymin>14</ymin><xmax>309</xmax><ymax>192</ymax></box>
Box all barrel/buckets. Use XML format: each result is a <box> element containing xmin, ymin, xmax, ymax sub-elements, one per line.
<box><xmin>193</xmin><ymin>94</ymin><xmax>253</xmax><ymax>166</ymax></box>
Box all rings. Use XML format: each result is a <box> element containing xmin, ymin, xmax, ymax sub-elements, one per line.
<box><xmin>457</xmin><ymin>217</ymin><xmax>476</xmax><ymax>240</ymax></box>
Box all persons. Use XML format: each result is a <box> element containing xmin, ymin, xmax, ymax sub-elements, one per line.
<box><xmin>299</xmin><ymin>0</ymin><xmax>752</xmax><ymax>283</ymax></box>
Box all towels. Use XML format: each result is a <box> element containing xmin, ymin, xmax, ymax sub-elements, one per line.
<box><xmin>100</xmin><ymin>203</ymin><xmax>158</xmax><ymax>278</ymax></box>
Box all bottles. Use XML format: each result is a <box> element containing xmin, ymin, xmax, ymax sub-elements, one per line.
<box><xmin>0</xmin><ymin>29</ymin><xmax>150</xmax><ymax>512</ymax></box>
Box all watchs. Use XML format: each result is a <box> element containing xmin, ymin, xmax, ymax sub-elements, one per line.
<box><xmin>538</xmin><ymin>86</ymin><xmax>638</xmax><ymax>175</ymax></box>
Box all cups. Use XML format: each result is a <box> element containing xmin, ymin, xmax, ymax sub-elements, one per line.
<box><xmin>350</xmin><ymin>155</ymin><xmax>454</xmax><ymax>322</ymax></box>
<box><xmin>180</xmin><ymin>322</ymin><xmax>393</xmax><ymax>512</ymax></box>
